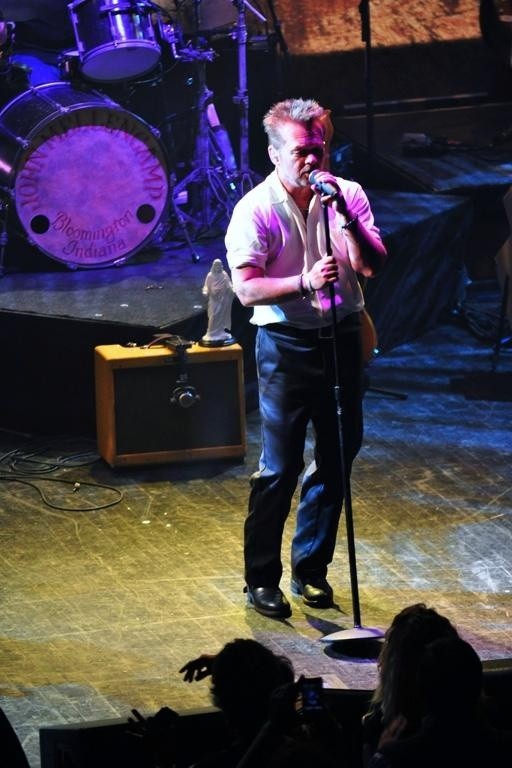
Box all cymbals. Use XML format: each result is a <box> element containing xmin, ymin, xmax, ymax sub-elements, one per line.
<box><xmin>191</xmin><ymin>0</ymin><xmax>239</xmax><ymax>33</ymax></box>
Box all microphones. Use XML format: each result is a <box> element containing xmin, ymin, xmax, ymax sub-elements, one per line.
<box><xmin>308</xmin><ymin>169</ymin><xmax>340</xmax><ymax>199</ymax></box>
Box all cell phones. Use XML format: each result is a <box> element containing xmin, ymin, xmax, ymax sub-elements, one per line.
<box><xmin>301</xmin><ymin>677</ymin><xmax>323</xmax><ymax>714</ymax></box>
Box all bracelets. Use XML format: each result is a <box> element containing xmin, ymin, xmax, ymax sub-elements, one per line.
<box><xmin>306</xmin><ymin>280</ymin><xmax>314</xmax><ymax>297</ymax></box>
<box><xmin>342</xmin><ymin>214</ymin><xmax>359</xmax><ymax>229</ymax></box>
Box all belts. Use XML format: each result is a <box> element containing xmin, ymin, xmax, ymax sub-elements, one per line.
<box><xmin>264</xmin><ymin>323</ymin><xmax>333</xmax><ymax>340</ymax></box>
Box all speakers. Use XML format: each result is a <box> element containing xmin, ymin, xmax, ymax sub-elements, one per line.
<box><xmin>94</xmin><ymin>341</ymin><xmax>247</xmax><ymax>469</ymax></box>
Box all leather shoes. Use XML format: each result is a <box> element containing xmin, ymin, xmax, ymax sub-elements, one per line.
<box><xmin>246</xmin><ymin>583</ymin><xmax>291</xmax><ymax>619</ymax></box>
<box><xmin>292</xmin><ymin>569</ymin><xmax>333</xmax><ymax>606</ymax></box>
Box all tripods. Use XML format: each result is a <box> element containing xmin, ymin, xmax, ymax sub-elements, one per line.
<box><xmin>172</xmin><ymin>60</ymin><xmax>238</xmax><ymax>229</ymax></box>
<box><xmin>190</xmin><ymin>12</ymin><xmax>265</xmax><ymax>242</ymax></box>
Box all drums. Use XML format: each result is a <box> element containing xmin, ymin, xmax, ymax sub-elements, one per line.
<box><xmin>62</xmin><ymin>50</ymin><xmax>81</xmax><ymax>82</ymax></box>
<box><xmin>0</xmin><ymin>82</ymin><xmax>172</xmax><ymax>269</ymax></box>
<box><xmin>69</xmin><ymin>1</ymin><xmax>160</xmax><ymax>83</ymax></box>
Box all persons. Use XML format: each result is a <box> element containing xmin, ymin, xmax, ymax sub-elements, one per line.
<box><xmin>225</xmin><ymin>96</ymin><xmax>388</xmax><ymax>619</ymax></box>
<box><xmin>200</xmin><ymin>258</ymin><xmax>234</xmax><ymax>341</ymax></box>
<box><xmin>123</xmin><ymin>605</ymin><xmax>512</xmax><ymax>768</ymax></box>
